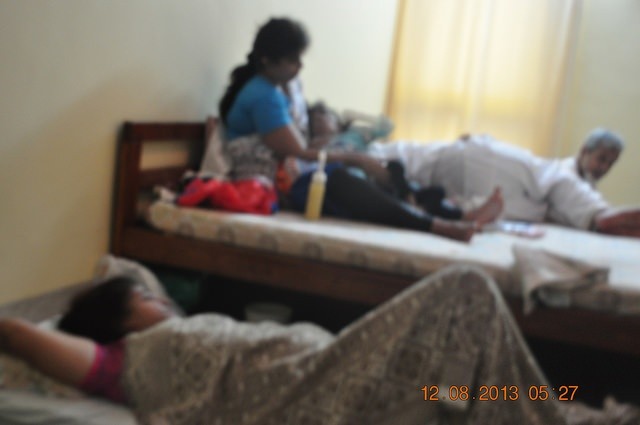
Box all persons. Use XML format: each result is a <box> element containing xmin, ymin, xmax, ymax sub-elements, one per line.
<box><xmin>558</xmin><ymin>130</ymin><xmax>623</xmax><ymax>186</ymax></box>
<box><xmin>0</xmin><ymin>262</ymin><xmax>568</xmax><ymax>425</ymax></box>
<box><xmin>307</xmin><ymin>102</ymin><xmax>640</xmax><ymax>239</ymax></box>
<box><xmin>219</xmin><ymin>16</ymin><xmax>505</xmax><ymax>242</ymax></box>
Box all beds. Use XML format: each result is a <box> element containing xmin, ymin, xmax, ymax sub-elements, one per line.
<box><xmin>1</xmin><ymin>280</ymin><xmax>157</xmax><ymax>425</ymax></box>
<box><xmin>116</xmin><ymin>120</ymin><xmax>640</xmax><ymax>401</ymax></box>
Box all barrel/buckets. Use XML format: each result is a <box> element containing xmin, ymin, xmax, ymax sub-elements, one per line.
<box><xmin>245</xmin><ymin>303</ymin><xmax>293</xmax><ymax>322</ymax></box>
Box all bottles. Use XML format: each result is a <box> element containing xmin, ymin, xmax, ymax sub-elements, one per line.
<box><xmin>306</xmin><ymin>150</ymin><xmax>328</xmax><ymax>218</ymax></box>
